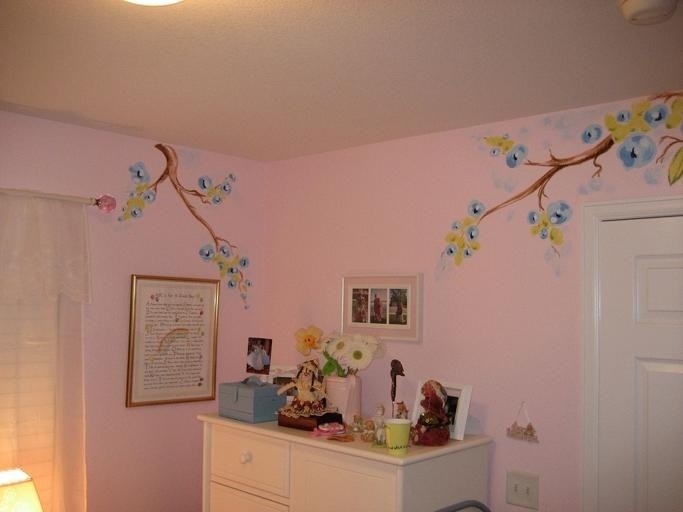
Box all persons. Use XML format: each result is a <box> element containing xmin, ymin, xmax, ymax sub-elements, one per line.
<box><xmin>354</xmin><ymin>294</ymin><xmax>368</xmax><ymax>323</ymax></box>
<box><xmin>373</xmin><ymin>293</ymin><xmax>382</xmax><ymax>322</ymax></box>
<box><xmin>395</xmin><ymin>299</ymin><xmax>406</xmax><ymax>323</ymax></box>
<box><xmin>246</xmin><ymin>338</ymin><xmax>270</xmax><ymax>373</ymax></box>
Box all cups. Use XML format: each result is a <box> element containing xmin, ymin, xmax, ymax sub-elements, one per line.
<box><xmin>384</xmin><ymin>419</ymin><xmax>412</xmax><ymax>456</ymax></box>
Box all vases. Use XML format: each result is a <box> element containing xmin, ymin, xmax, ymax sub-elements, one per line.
<box><xmin>324</xmin><ymin>376</ymin><xmax>361</xmax><ymax>426</ymax></box>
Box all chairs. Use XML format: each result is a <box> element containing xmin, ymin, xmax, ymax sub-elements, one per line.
<box><xmin>436</xmin><ymin>499</ymin><xmax>492</xmax><ymax>512</ymax></box>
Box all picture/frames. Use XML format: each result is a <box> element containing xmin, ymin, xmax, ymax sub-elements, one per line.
<box><xmin>413</xmin><ymin>381</ymin><xmax>472</xmax><ymax>441</ymax></box>
<box><xmin>339</xmin><ymin>272</ymin><xmax>422</xmax><ymax>344</ymax></box>
<box><xmin>125</xmin><ymin>273</ymin><xmax>221</xmax><ymax>409</ymax></box>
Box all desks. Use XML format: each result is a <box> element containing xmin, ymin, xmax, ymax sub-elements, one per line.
<box><xmin>197</xmin><ymin>411</ymin><xmax>496</xmax><ymax>512</ymax></box>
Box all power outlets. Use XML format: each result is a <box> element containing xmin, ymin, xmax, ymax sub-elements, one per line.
<box><xmin>505</xmin><ymin>468</ymin><xmax>540</xmax><ymax>510</ymax></box>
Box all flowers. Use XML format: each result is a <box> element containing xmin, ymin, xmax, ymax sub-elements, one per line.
<box><xmin>292</xmin><ymin>322</ymin><xmax>380</xmax><ymax>377</ymax></box>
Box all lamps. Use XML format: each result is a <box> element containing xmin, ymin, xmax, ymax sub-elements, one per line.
<box><xmin>0</xmin><ymin>468</ymin><xmax>45</xmax><ymax>512</ymax></box>
